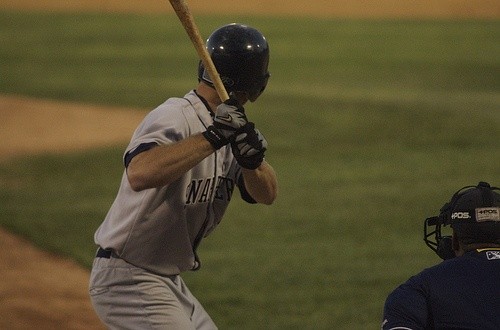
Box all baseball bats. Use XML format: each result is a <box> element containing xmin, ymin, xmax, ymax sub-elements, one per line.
<box><xmin>170</xmin><ymin>0</ymin><xmax>231</xmax><ymax>103</ymax></box>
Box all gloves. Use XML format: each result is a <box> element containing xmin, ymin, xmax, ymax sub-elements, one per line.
<box><xmin>228</xmin><ymin>122</ymin><xmax>269</xmax><ymax>170</ymax></box>
<box><xmin>201</xmin><ymin>98</ymin><xmax>248</xmax><ymax>151</ymax></box>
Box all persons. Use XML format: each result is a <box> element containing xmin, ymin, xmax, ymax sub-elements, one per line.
<box><xmin>382</xmin><ymin>182</ymin><xmax>500</xmax><ymax>330</ymax></box>
<box><xmin>88</xmin><ymin>23</ymin><xmax>276</xmax><ymax>330</ymax></box>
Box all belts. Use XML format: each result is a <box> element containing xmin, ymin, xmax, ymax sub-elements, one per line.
<box><xmin>96</xmin><ymin>247</ymin><xmax>112</xmax><ymax>259</ymax></box>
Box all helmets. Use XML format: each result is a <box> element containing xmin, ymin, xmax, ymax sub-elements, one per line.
<box><xmin>197</xmin><ymin>22</ymin><xmax>271</xmax><ymax>102</ymax></box>
<box><xmin>424</xmin><ymin>180</ymin><xmax>500</xmax><ymax>259</ymax></box>
